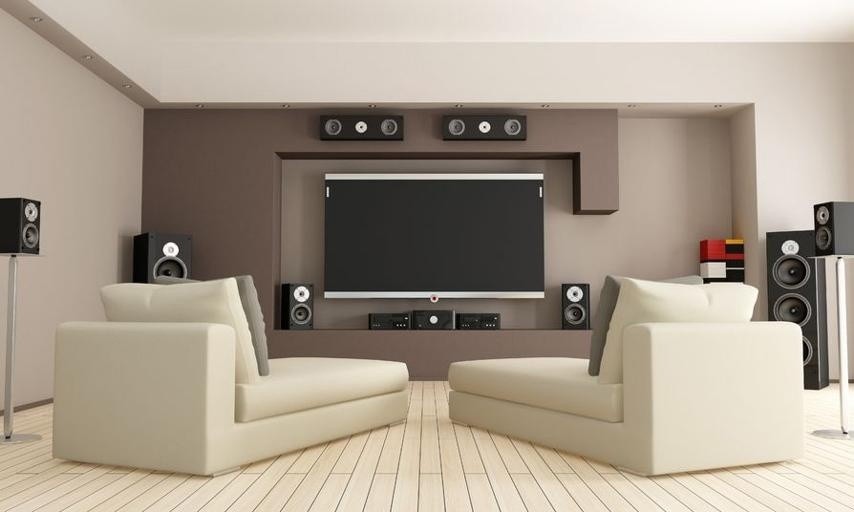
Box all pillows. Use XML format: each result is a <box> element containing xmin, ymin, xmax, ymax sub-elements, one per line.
<box><xmin>586</xmin><ymin>273</ymin><xmax>758</xmax><ymax>379</ymax></box>
<box><xmin>97</xmin><ymin>274</ymin><xmax>269</xmax><ymax>386</ymax></box>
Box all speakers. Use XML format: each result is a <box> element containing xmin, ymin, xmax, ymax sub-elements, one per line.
<box><xmin>281</xmin><ymin>283</ymin><xmax>313</xmax><ymax>330</ymax></box>
<box><xmin>766</xmin><ymin>230</ymin><xmax>829</xmax><ymax>389</ymax></box>
<box><xmin>562</xmin><ymin>284</ymin><xmax>590</xmax><ymax>330</ymax></box>
<box><xmin>320</xmin><ymin>114</ymin><xmax>404</xmax><ymax>141</ymax></box>
<box><xmin>0</xmin><ymin>198</ymin><xmax>40</xmax><ymax>254</ymax></box>
<box><xmin>814</xmin><ymin>201</ymin><xmax>854</xmax><ymax>256</ymax></box>
<box><xmin>444</xmin><ymin>115</ymin><xmax>526</xmax><ymax>140</ymax></box>
<box><xmin>133</xmin><ymin>232</ymin><xmax>192</xmax><ymax>283</ymax></box>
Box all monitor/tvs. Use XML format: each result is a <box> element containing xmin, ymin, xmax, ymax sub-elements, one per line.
<box><xmin>324</xmin><ymin>174</ymin><xmax>544</xmax><ymax>298</ymax></box>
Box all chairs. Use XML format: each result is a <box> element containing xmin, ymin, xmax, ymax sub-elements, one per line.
<box><xmin>53</xmin><ymin>318</ymin><xmax>408</xmax><ymax>478</ymax></box>
<box><xmin>442</xmin><ymin>320</ymin><xmax>808</xmax><ymax>479</ymax></box>
